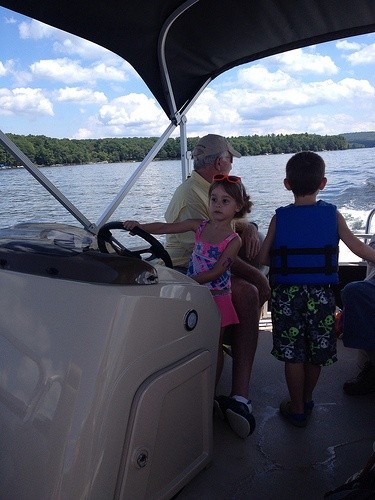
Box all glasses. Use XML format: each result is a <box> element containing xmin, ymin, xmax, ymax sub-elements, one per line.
<box><xmin>212</xmin><ymin>174</ymin><xmax>244</xmax><ymax>200</ymax></box>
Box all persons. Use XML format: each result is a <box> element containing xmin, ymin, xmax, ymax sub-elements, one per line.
<box><xmin>260</xmin><ymin>153</ymin><xmax>375</xmax><ymax>424</ymax></box>
<box><xmin>164</xmin><ymin>134</ymin><xmax>272</xmax><ymax>437</ymax></box>
<box><xmin>123</xmin><ymin>174</ymin><xmax>252</xmax><ymax>415</ymax></box>
<box><xmin>340</xmin><ymin>234</ymin><xmax>375</xmax><ymax>395</ymax></box>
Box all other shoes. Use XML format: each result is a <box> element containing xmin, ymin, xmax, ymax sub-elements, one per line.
<box><xmin>279</xmin><ymin>397</ymin><xmax>315</xmax><ymax>429</ymax></box>
<box><xmin>214</xmin><ymin>395</ymin><xmax>257</xmax><ymax>440</ymax></box>
<box><xmin>343</xmin><ymin>364</ymin><xmax>375</xmax><ymax>395</ymax></box>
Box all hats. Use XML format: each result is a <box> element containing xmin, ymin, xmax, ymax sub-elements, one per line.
<box><xmin>190</xmin><ymin>133</ymin><xmax>242</xmax><ymax>161</ymax></box>
